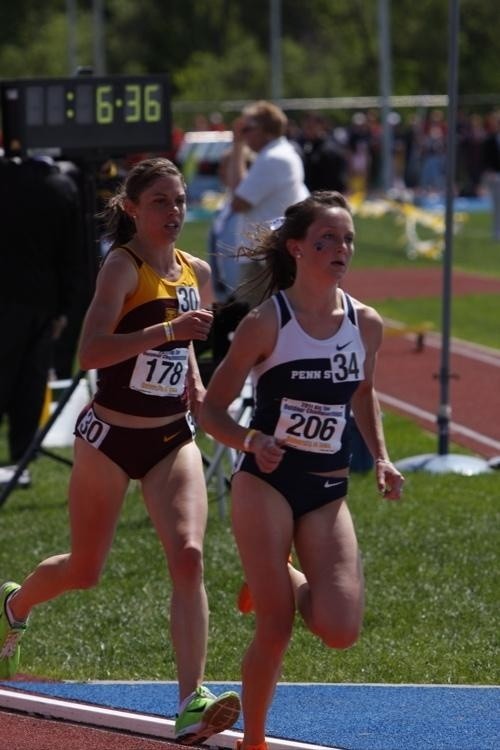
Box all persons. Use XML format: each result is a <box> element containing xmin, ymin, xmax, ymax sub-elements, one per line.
<box><xmin>2</xmin><ymin>152</ymin><xmax>240</xmax><ymax>749</ymax></box>
<box><xmin>193</xmin><ymin>187</ymin><xmax>407</xmax><ymax>749</ymax></box>
<box><xmin>204</xmin><ymin>143</ymin><xmax>255</xmax><ymax>307</ymax></box>
<box><xmin>192</xmin><ymin>106</ymin><xmax>499</xmax><ymax>198</ymax></box>
<box><xmin>2</xmin><ymin>138</ymin><xmax>119</xmax><ymax>488</ymax></box>
<box><xmin>225</xmin><ymin>101</ymin><xmax>308</xmax><ymax>305</ymax></box>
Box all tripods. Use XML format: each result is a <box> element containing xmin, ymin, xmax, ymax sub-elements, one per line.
<box><xmin>199</xmin><ymin>401</ymin><xmax>256</xmax><ymax>528</ymax></box>
<box><xmin>1</xmin><ymin>371</ymin><xmax>92</xmax><ymax>510</ymax></box>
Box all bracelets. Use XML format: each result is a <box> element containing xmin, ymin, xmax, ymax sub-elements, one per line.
<box><xmin>376</xmin><ymin>459</ymin><xmax>392</xmax><ymax>466</ymax></box>
<box><xmin>243</xmin><ymin>428</ymin><xmax>261</xmax><ymax>451</ymax></box>
<box><xmin>161</xmin><ymin>320</ymin><xmax>175</xmax><ymax>342</ymax></box>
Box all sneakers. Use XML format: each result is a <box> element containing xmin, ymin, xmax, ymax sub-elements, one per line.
<box><xmin>0</xmin><ymin>582</ymin><xmax>27</xmax><ymax>664</ymax></box>
<box><xmin>174</xmin><ymin>686</ymin><xmax>242</xmax><ymax>744</ymax></box>
<box><xmin>238</xmin><ymin>554</ymin><xmax>293</xmax><ymax>611</ymax></box>
